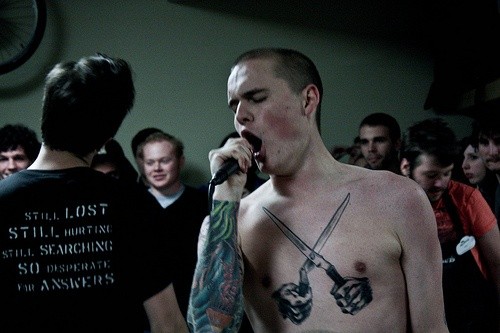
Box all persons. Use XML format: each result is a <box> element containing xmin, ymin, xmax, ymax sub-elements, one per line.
<box><xmin>0</xmin><ymin>53</ymin><xmax>188</xmax><ymax>333</ymax></box>
<box><xmin>90</xmin><ymin>127</ymin><xmax>266</xmax><ymax>319</ymax></box>
<box><xmin>333</xmin><ymin>113</ymin><xmax>500</xmax><ymax>333</ymax></box>
<box><xmin>187</xmin><ymin>48</ymin><xmax>450</xmax><ymax>333</ymax></box>
<box><xmin>0</xmin><ymin>124</ymin><xmax>41</xmax><ymax>182</ymax></box>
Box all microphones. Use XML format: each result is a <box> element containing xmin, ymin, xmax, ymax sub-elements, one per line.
<box><xmin>210</xmin><ymin>157</ymin><xmax>239</xmax><ymax>185</ymax></box>
